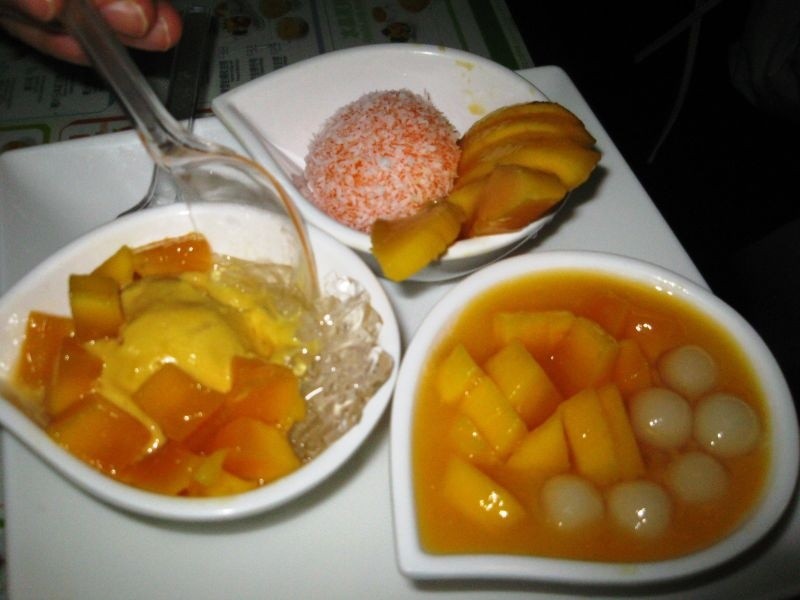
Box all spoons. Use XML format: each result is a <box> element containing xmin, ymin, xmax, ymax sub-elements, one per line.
<box><xmin>48</xmin><ymin>0</ymin><xmax>324</xmax><ymax>306</ymax></box>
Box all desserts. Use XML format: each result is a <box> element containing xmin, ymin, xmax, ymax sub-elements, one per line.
<box><xmin>302</xmin><ymin>89</ymin><xmax>461</xmax><ymax>231</ymax></box>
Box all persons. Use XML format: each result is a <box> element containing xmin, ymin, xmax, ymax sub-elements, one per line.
<box><xmin>0</xmin><ymin>0</ymin><xmax>183</xmax><ymax>66</ymax></box>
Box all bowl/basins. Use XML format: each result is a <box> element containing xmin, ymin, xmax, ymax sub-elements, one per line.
<box><xmin>391</xmin><ymin>249</ymin><xmax>800</xmax><ymax>583</ymax></box>
<box><xmin>214</xmin><ymin>42</ymin><xmax>568</xmax><ymax>285</ymax></box>
<box><xmin>0</xmin><ymin>200</ymin><xmax>401</xmax><ymax>524</ymax></box>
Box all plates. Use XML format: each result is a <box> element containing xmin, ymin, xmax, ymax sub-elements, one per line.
<box><xmin>1</xmin><ymin>63</ymin><xmax>799</xmax><ymax>599</ymax></box>
